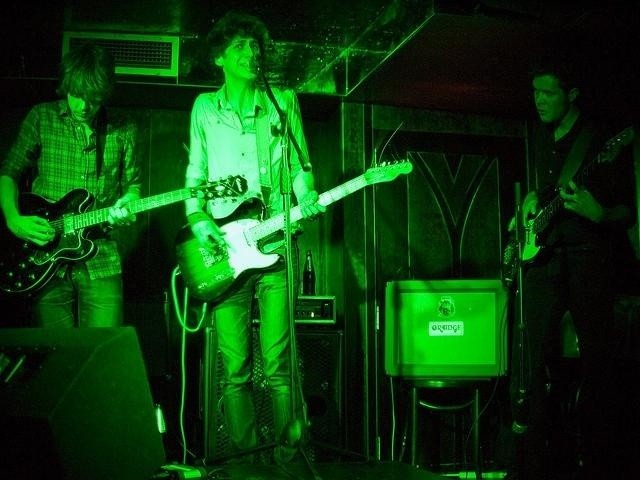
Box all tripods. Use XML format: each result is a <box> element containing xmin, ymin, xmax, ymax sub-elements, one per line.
<box><xmin>184</xmin><ymin>74</ymin><xmax>379</xmax><ymax>480</ymax></box>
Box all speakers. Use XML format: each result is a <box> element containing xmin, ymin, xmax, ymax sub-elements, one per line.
<box><xmin>0</xmin><ymin>326</ymin><xmax>167</xmax><ymax>480</ymax></box>
<box><xmin>201</xmin><ymin>323</ymin><xmax>346</xmax><ymax>462</ymax></box>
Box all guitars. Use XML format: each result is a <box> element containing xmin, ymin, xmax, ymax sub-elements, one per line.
<box><xmin>176</xmin><ymin>159</ymin><xmax>414</xmax><ymax>301</ymax></box>
<box><xmin>1</xmin><ymin>175</ymin><xmax>247</xmax><ymax>296</ymax></box>
<box><xmin>504</xmin><ymin>129</ymin><xmax>635</xmax><ymax>264</ymax></box>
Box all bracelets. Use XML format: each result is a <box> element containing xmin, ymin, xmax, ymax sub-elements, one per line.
<box><xmin>185</xmin><ymin>213</ymin><xmax>214</xmax><ymax>224</ymax></box>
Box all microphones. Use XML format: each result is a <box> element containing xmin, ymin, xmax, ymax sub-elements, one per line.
<box><xmin>511</xmin><ymin>383</ymin><xmax>533</xmax><ymax>435</ymax></box>
<box><xmin>515</xmin><ymin>182</ymin><xmax>524</xmax><ymax>243</ymax></box>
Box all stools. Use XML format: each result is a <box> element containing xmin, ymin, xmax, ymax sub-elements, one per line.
<box><xmin>408</xmin><ymin>377</ymin><xmax>489</xmax><ymax>479</ymax></box>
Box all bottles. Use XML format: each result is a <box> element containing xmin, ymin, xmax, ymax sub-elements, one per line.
<box><xmin>302</xmin><ymin>248</ymin><xmax>317</xmax><ymax>296</ymax></box>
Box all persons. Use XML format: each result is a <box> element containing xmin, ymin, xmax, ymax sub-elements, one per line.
<box><xmin>502</xmin><ymin>64</ymin><xmax>637</xmax><ymax>480</ymax></box>
<box><xmin>182</xmin><ymin>9</ymin><xmax>329</xmax><ymax>461</ymax></box>
<box><xmin>1</xmin><ymin>43</ymin><xmax>145</xmax><ymax>331</ymax></box>
